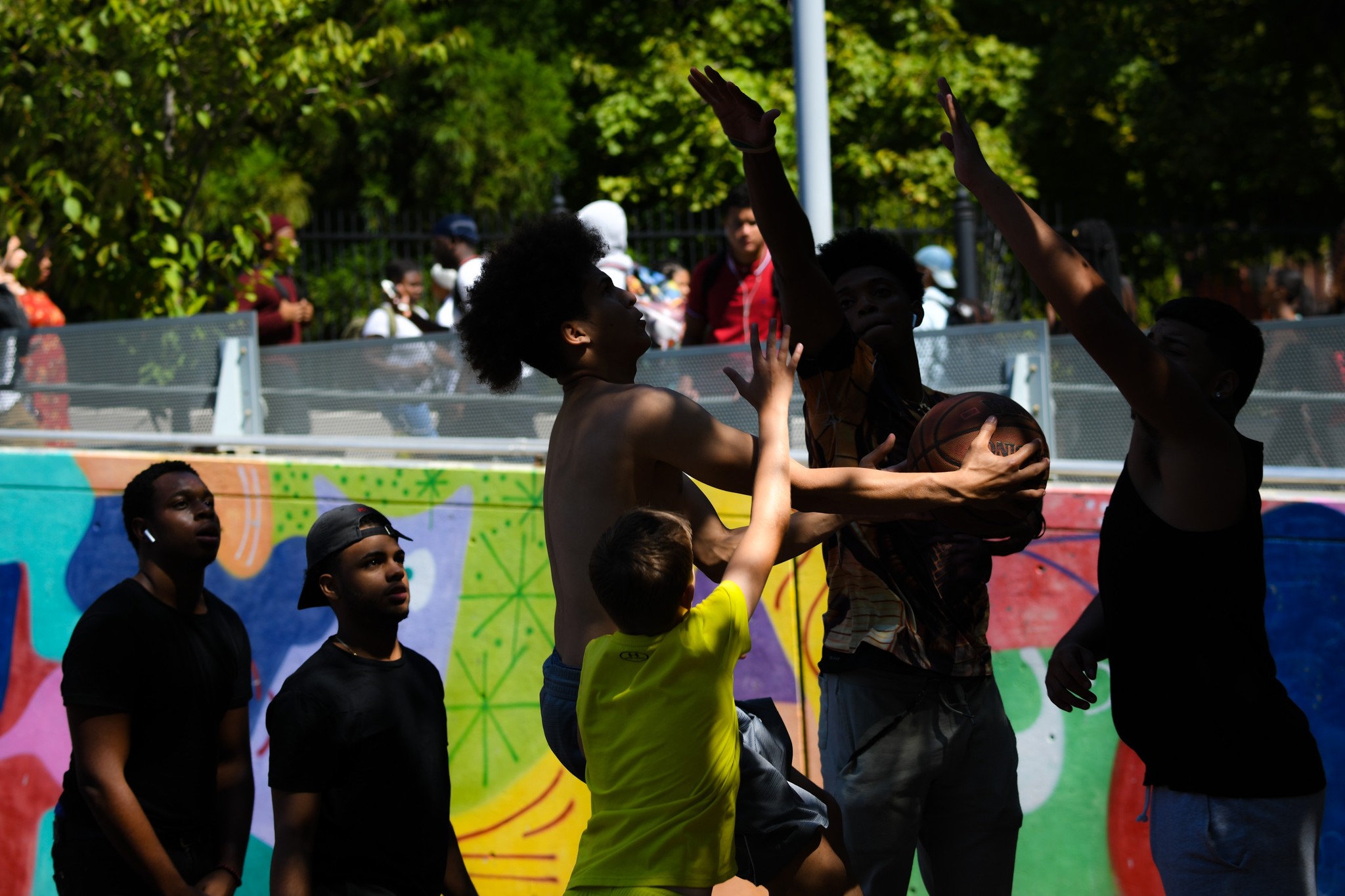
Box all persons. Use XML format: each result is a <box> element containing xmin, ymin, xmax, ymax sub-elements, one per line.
<box><xmin>224</xmin><ymin>194</ymin><xmax>1345</xmax><ymax>466</ymax></box>
<box><xmin>52</xmin><ymin>461</ymin><xmax>256</xmax><ymax>895</ymax></box>
<box><xmin>267</xmin><ymin>504</ymin><xmax>480</xmax><ymax>896</ymax></box>
<box><xmin>455</xmin><ymin>66</ymin><xmax>1051</xmax><ymax>896</ymax></box>
<box><xmin>931</xmin><ymin>75</ymin><xmax>1326</xmax><ymax>896</ymax></box>
<box><xmin>0</xmin><ymin>234</ymin><xmax>69</xmax><ymax>445</ymax></box>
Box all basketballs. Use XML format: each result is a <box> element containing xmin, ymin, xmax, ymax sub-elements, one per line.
<box><xmin>907</xmin><ymin>392</ymin><xmax>1049</xmax><ymax>536</ymax></box>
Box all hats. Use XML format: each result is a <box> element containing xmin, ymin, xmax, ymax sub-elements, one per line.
<box><xmin>298</xmin><ymin>504</ymin><xmax>414</xmax><ymax>610</ymax></box>
<box><xmin>430</xmin><ymin>213</ymin><xmax>480</xmax><ymax>243</ymax></box>
<box><xmin>253</xmin><ymin>215</ymin><xmax>291</xmax><ymax>241</ymax></box>
<box><xmin>429</xmin><ymin>263</ymin><xmax>458</xmax><ymax>291</ymax></box>
<box><xmin>914</xmin><ymin>244</ymin><xmax>958</xmax><ymax>288</ymax></box>
